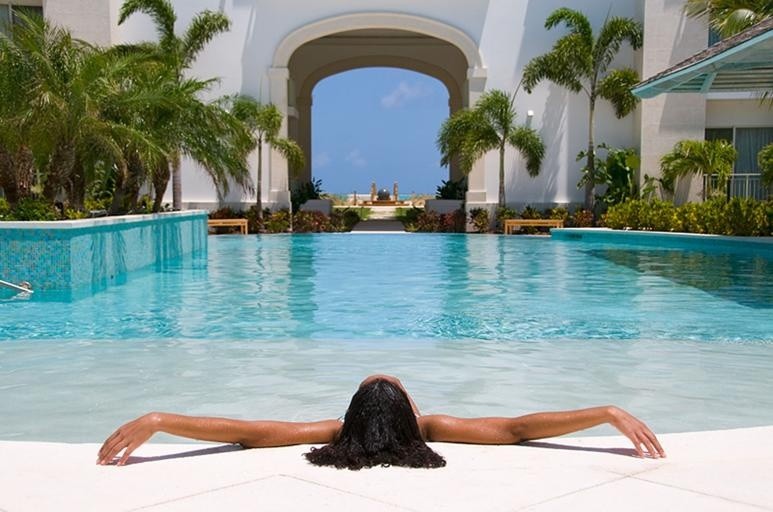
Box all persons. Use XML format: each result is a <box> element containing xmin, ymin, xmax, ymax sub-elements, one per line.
<box><xmin>94</xmin><ymin>374</ymin><xmax>665</xmax><ymax>471</ymax></box>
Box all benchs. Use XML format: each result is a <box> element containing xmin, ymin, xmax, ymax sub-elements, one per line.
<box><xmin>504</xmin><ymin>219</ymin><xmax>564</xmax><ymax>235</ymax></box>
<box><xmin>208</xmin><ymin>218</ymin><xmax>249</xmax><ymax>234</ymax></box>
